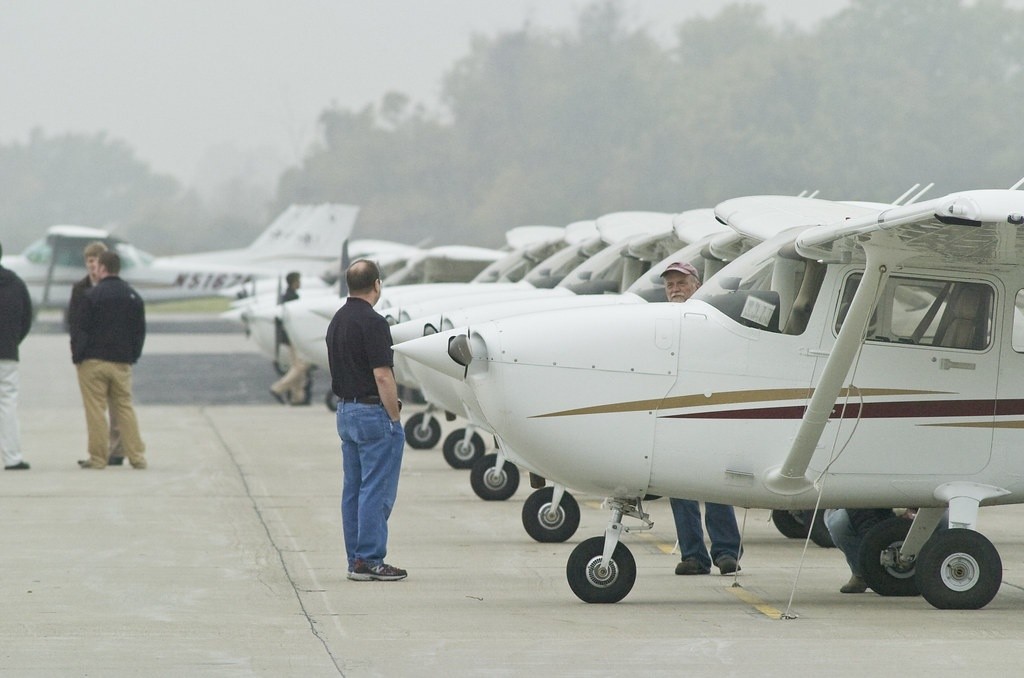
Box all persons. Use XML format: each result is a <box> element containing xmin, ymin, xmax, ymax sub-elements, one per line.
<box><xmin>0</xmin><ymin>243</ymin><xmax>33</xmax><ymax>470</ymax></box>
<box><xmin>659</xmin><ymin>263</ymin><xmax>745</xmax><ymax>574</ymax></box>
<box><xmin>270</xmin><ymin>272</ymin><xmax>315</xmax><ymax>405</ymax></box>
<box><xmin>827</xmin><ymin>507</ymin><xmax>949</xmax><ymax>594</ymax></box>
<box><xmin>326</xmin><ymin>260</ymin><xmax>409</xmax><ymax>582</ymax></box>
<box><xmin>69</xmin><ymin>252</ymin><xmax>148</xmax><ymax>469</ymax></box>
<box><xmin>70</xmin><ymin>240</ymin><xmax>125</xmax><ymax>466</ymax></box>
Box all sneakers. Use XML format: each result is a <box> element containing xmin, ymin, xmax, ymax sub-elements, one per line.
<box><xmin>675</xmin><ymin>560</ymin><xmax>709</xmax><ymax>575</ymax></box>
<box><xmin>718</xmin><ymin>557</ymin><xmax>741</xmax><ymax>574</ymax></box>
<box><xmin>347</xmin><ymin>564</ymin><xmax>407</xmax><ymax>580</ymax></box>
<box><xmin>840</xmin><ymin>573</ymin><xmax>869</xmax><ymax>593</ymax></box>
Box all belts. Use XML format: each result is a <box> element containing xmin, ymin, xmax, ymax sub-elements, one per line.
<box><xmin>340</xmin><ymin>398</ymin><xmax>383</xmax><ymax>404</ymax></box>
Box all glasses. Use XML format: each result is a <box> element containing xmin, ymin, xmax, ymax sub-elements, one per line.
<box><xmin>377</xmin><ymin>277</ymin><xmax>384</xmax><ymax>284</ymax></box>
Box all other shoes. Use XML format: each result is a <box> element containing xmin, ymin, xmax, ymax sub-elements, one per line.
<box><xmin>292</xmin><ymin>400</ymin><xmax>309</xmax><ymax>406</ymax></box>
<box><xmin>78</xmin><ymin>460</ymin><xmax>104</xmax><ymax>470</ymax></box>
<box><xmin>108</xmin><ymin>456</ymin><xmax>123</xmax><ymax>466</ymax></box>
<box><xmin>270</xmin><ymin>388</ymin><xmax>286</xmax><ymax>403</ymax></box>
<box><xmin>5</xmin><ymin>462</ymin><xmax>30</xmax><ymax>471</ymax></box>
<box><xmin>134</xmin><ymin>461</ymin><xmax>146</xmax><ymax>469</ymax></box>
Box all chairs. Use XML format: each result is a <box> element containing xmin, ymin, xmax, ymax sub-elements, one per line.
<box><xmin>934</xmin><ymin>284</ymin><xmax>992</xmax><ymax>349</ymax></box>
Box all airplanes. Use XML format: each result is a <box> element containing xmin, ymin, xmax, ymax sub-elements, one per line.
<box><xmin>0</xmin><ymin>204</ymin><xmax>420</xmax><ymax>312</ymax></box>
<box><xmin>220</xmin><ymin>179</ymin><xmax>1024</xmax><ymax>610</ymax></box>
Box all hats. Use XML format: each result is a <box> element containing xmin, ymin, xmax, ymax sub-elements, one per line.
<box><xmin>660</xmin><ymin>262</ymin><xmax>699</xmax><ymax>277</ymax></box>
